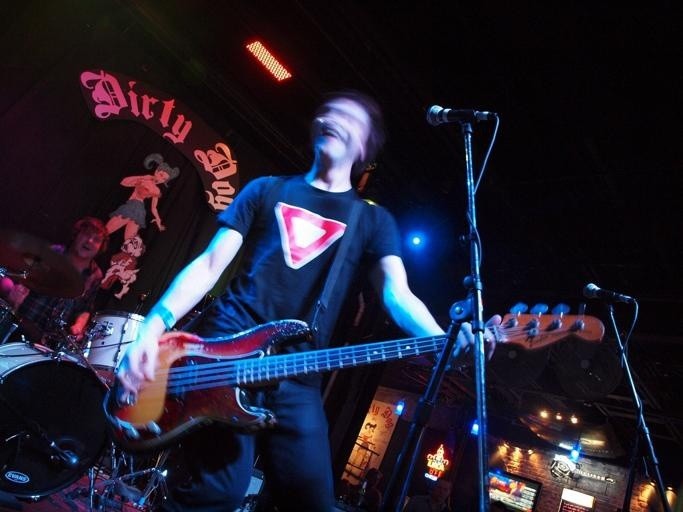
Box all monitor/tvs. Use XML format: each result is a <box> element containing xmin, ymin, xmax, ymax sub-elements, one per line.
<box><xmin>489</xmin><ymin>465</ymin><xmax>545</xmax><ymax>512</ymax></box>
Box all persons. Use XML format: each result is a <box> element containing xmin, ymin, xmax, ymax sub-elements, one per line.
<box><xmin>5</xmin><ymin>213</ymin><xmax>109</xmax><ymax>346</ymax></box>
<box><xmin>103</xmin><ymin>86</ymin><xmax>502</xmax><ymax>510</ymax></box>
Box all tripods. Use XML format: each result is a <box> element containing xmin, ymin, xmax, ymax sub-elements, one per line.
<box><xmin>66</xmin><ymin>447</ymin><xmax>171</xmax><ymax>512</ymax></box>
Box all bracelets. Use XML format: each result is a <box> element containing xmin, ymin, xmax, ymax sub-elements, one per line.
<box><xmin>151</xmin><ymin>303</ymin><xmax>176</xmax><ymax>332</ymax></box>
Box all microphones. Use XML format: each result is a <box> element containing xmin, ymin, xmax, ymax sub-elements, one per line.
<box><xmin>582</xmin><ymin>282</ymin><xmax>637</xmax><ymax>304</ymax></box>
<box><xmin>425</xmin><ymin>103</ymin><xmax>499</xmax><ymax>126</ymax></box>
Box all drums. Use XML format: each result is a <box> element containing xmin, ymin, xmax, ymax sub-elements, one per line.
<box><xmin>84</xmin><ymin>312</ymin><xmax>146</xmax><ymax>384</ymax></box>
<box><xmin>1</xmin><ymin>343</ymin><xmax>110</xmax><ymax>499</ymax></box>
<box><xmin>0</xmin><ymin>300</ymin><xmax>26</xmax><ymax>346</ymax></box>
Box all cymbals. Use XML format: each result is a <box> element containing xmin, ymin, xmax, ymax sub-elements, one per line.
<box><xmin>0</xmin><ymin>231</ymin><xmax>84</xmax><ymax>297</ymax></box>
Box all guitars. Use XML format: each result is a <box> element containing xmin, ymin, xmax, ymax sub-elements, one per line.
<box><xmin>103</xmin><ymin>304</ymin><xmax>604</xmax><ymax>457</ymax></box>
